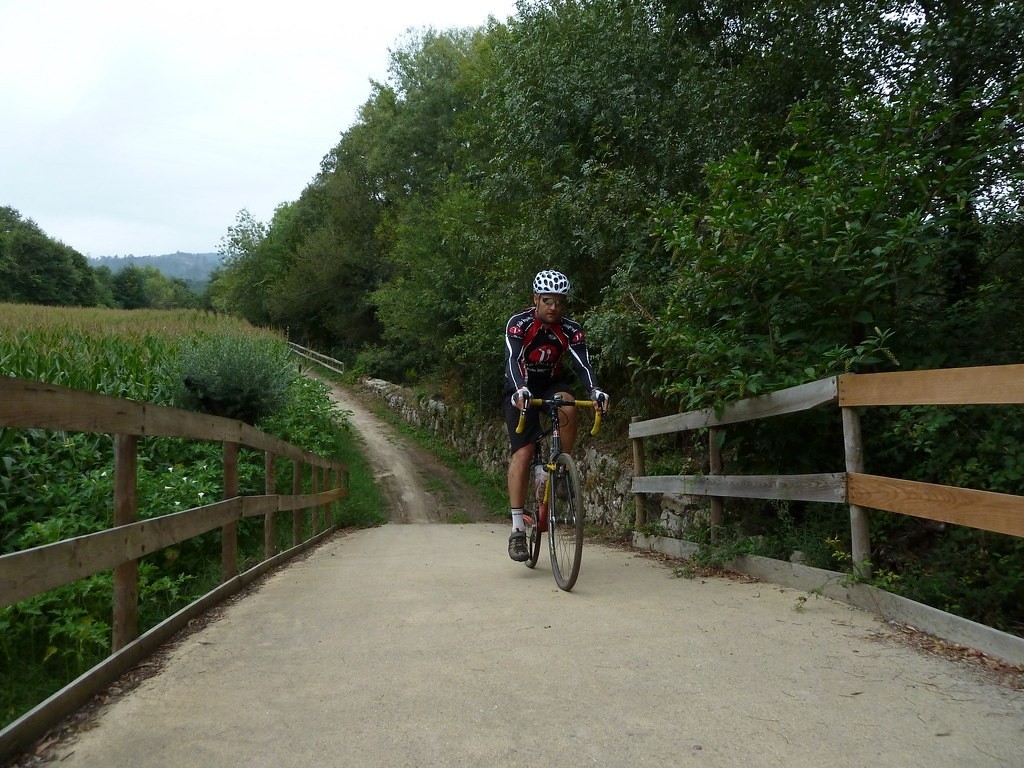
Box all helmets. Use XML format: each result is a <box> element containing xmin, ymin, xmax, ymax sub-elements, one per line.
<box><xmin>533</xmin><ymin>270</ymin><xmax>570</xmax><ymax>296</ymax></box>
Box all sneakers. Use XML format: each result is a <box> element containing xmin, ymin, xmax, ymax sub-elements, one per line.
<box><xmin>508</xmin><ymin>531</ymin><xmax>530</xmax><ymax>562</ymax></box>
<box><xmin>557</xmin><ymin>476</ymin><xmax>575</xmax><ymax>499</ymax></box>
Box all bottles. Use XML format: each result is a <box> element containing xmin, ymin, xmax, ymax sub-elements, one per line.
<box><xmin>534</xmin><ymin>462</ymin><xmax>544</xmax><ymax>493</ymax></box>
<box><xmin>536</xmin><ymin>466</ymin><xmax>550</xmax><ymax>503</ymax></box>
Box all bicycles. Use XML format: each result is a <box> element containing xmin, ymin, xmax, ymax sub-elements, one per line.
<box><xmin>511</xmin><ymin>390</ymin><xmax>607</xmax><ymax>591</ymax></box>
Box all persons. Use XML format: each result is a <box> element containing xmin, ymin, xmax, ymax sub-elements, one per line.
<box><xmin>504</xmin><ymin>270</ymin><xmax>610</xmax><ymax>562</ymax></box>
